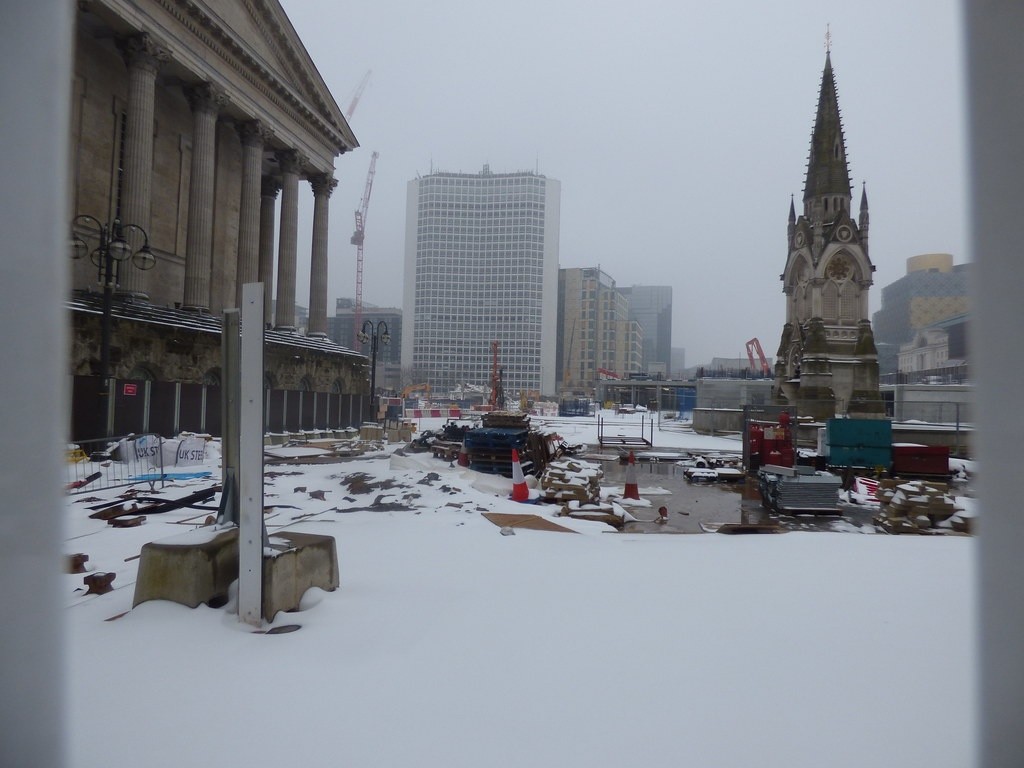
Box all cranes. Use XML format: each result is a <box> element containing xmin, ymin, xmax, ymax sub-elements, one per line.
<box><xmin>343</xmin><ymin>67</ymin><xmax>374</xmax><ymax>124</ymax></box>
<box><xmin>351</xmin><ymin>152</ymin><xmax>379</xmax><ymax>351</ymax></box>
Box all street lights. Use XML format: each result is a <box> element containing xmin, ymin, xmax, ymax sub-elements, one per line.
<box><xmin>357</xmin><ymin>319</ymin><xmax>392</xmax><ymax>425</ymax></box>
<box><xmin>69</xmin><ymin>215</ymin><xmax>158</xmax><ymax>452</ymax></box>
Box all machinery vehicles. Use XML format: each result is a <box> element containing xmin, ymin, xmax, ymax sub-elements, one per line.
<box><xmin>518</xmin><ymin>390</ymin><xmax>535</xmax><ymax>412</ymax></box>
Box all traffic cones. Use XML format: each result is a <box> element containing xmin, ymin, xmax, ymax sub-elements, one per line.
<box><xmin>623</xmin><ymin>450</ymin><xmax>641</xmax><ymax>500</ymax></box>
<box><xmin>507</xmin><ymin>448</ymin><xmax>530</xmax><ymax>502</ymax></box>
